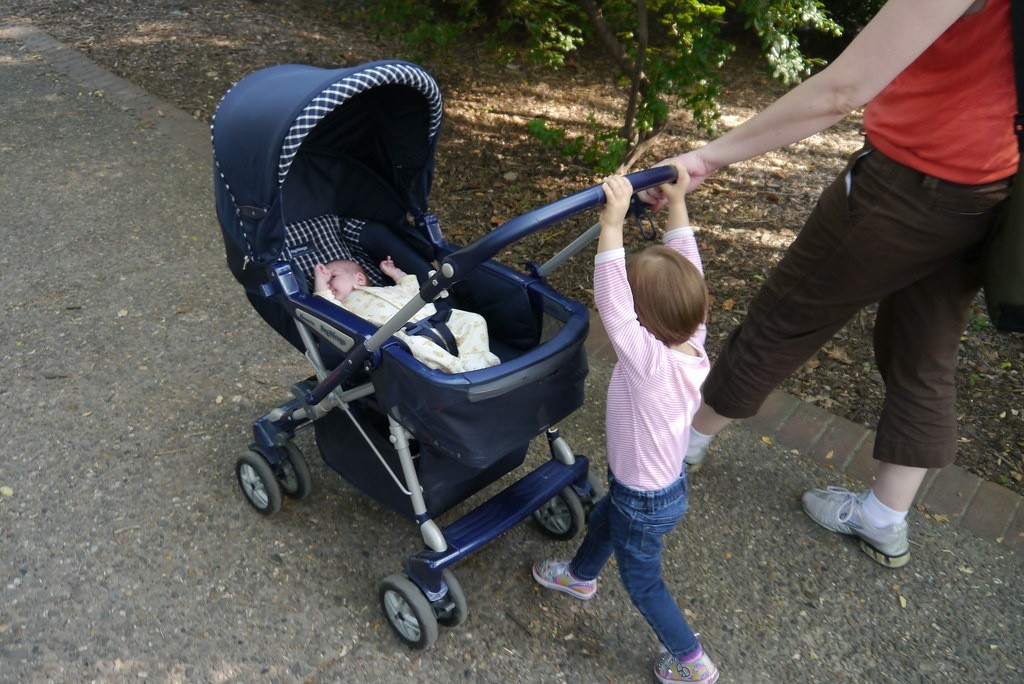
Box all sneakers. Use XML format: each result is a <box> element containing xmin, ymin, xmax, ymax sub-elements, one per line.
<box><xmin>653</xmin><ymin>651</ymin><xmax>720</xmax><ymax>684</ymax></box>
<box><xmin>800</xmin><ymin>485</ymin><xmax>913</xmax><ymax>568</ymax></box>
<box><xmin>532</xmin><ymin>558</ymin><xmax>598</xmax><ymax>600</ymax></box>
<box><xmin>683</xmin><ymin>460</ymin><xmax>705</xmax><ymax>472</ymax></box>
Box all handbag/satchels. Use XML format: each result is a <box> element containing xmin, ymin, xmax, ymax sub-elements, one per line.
<box><xmin>981</xmin><ymin>174</ymin><xmax>1024</xmax><ymax>332</ymax></box>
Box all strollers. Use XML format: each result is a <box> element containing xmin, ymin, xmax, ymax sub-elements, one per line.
<box><xmin>208</xmin><ymin>57</ymin><xmax>681</xmax><ymax>653</ymax></box>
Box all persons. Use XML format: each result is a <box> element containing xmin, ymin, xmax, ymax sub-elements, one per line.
<box><xmin>313</xmin><ymin>256</ymin><xmax>501</xmax><ymax>374</ymax></box>
<box><xmin>531</xmin><ymin>162</ymin><xmax>721</xmax><ymax>684</ymax></box>
<box><xmin>639</xmin><ymin>0</ymin><xmax>1024</xmax><ymax>567</ymax></box>
